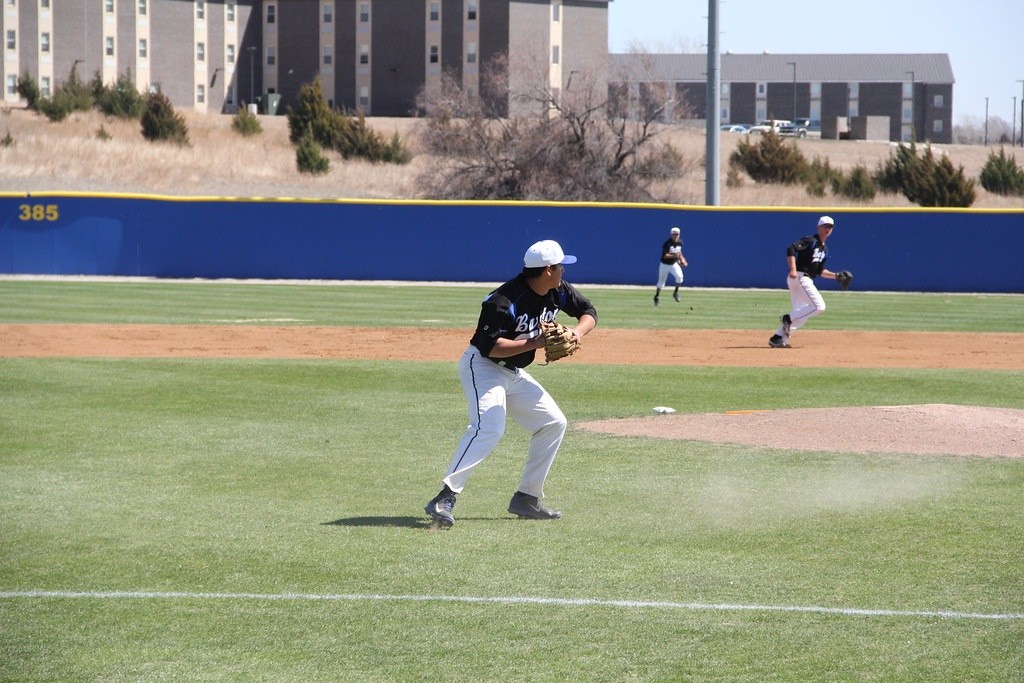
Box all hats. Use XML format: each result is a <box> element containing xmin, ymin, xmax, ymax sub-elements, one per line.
<box><xmin>670</xmin><ymin>227</ymin><xmax>681</xmax><ymax>235</ymax></box>
<box><xmin>817</xmin><ymin>216</ymin><xmax>835</xmax><ymax>226</ymax></box>
<box><xmin>523</xmin><ymin>240</ymin><xmax>578</xmax><ymax>268</ymax></box>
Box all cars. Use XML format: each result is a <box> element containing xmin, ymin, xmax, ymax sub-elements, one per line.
<box><xmin>721</xmin><ymin>123</ymin><xmax>755</xmax><ymax>132</ymax></box>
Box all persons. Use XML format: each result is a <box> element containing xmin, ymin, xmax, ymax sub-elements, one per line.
<box><xmin>767</xmin><ymin>215</ymin><xmax>853</xmax><ymax>348</ymax></box>
<box><xmin>423</xmin><ymin>240</ymin><xmax>598</xmax><ymax>529</ymax></box>
<box><xmin>653</xmin><ymin>227</ymin><xmax>688</xmax><ymax>306</ymax></box>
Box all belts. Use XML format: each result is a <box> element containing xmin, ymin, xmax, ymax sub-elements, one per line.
<box><xmin>803</xmin><ymin>272</ymin><xmax>813</xmax><ymax>279</ymax></box>
<box><xmin>476</xmin><ymin>346</ymin><xmax>516</xmax><ymax>371</ymax></box>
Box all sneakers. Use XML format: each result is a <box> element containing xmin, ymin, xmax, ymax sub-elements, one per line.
<box><xmin>508</xmin><ymin>492</ymin><xmax>562</xmax><ymax>520</ymax></box>
<box><xmin>653</xmin><ymin>295</ymin><xmax>659</xmax><ymax>304</ymax></box>
<box><xmin>781</xmin><ymin>315</ymin><xmax>791</xmax><ymax>338</ymax></box>
<box><xmin>425</xmin><ymin>490</ymin><xmax>455</xmax><ymax>527</ymax></box>
<box><xmin>673</xmin><ymin>293</ymin><xmax>680</xmax><ymax>302</ymax></box>
<box><xmin>768</xmin><ymin>334</ymin><xmax>792</xmax><ymax>348</ymax></box>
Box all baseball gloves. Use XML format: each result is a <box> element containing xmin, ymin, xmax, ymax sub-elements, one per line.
<box><xmin>538</xmin><ymin>319</ymin><xmax>582</xmax><ymax>367</ymax></box>
<box><xmin>836</xmin><ymin>270</ymin><xmax>853</xmax><ymax>288</ymax></box>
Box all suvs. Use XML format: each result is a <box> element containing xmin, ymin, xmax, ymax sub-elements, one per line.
<box><xmin>748</xmin><ymin>119</ymin><xmax>792</xmax><ymax>135</ymax></box>
<box><xmin>777</xmin><ymin>117</ymin><xmax>821</xmax><ymax>139</ymax></box>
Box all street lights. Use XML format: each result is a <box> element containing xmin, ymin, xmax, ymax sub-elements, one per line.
<box><xmin>1015</xmin><ymin>79</ymin><xmax>1024</xmax><ymax>147</ymax></box>
<box><xmin>247</xmin><ymin>46</ymin><xmax>256</xmax><ymax>104</ymax></box>
<box><xmin>786</xmin><ymin>62</ymin><xmax>797</xmax><ymax>124</ymax></box>
<box><xmin>1012</xmin><ymin>96</ymin><xmax>1016</xmax><ymax>147</ymax></box>
<box><xmin>905</xmin><ymin>71</ymin><xmax>914</xmax><ymax>143</ymax></box>
<box><xmin>984</xmin><ymin>97</ymin><xmax>988</xmax><ymax>146</ymax></box>
